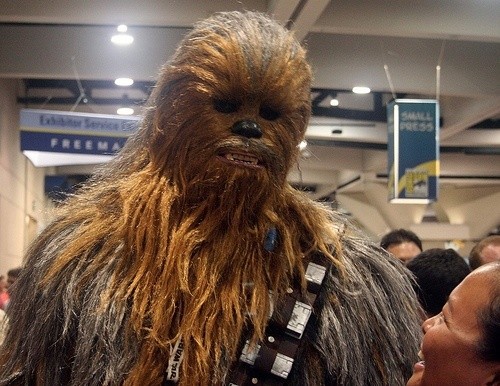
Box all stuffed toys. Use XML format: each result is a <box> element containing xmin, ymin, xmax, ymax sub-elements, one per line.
<box><xmin>0</xmin><ymin>10</ymin><xmax>427</xmax><ymax>386</ymax></box>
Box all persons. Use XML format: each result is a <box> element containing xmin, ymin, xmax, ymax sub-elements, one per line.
<box><xmin>406</xmin><ymin>261</ymin><xmax>500</xmax><ymax>386</ymax></box>
<box><xmin>0</xmin><ymin>268</ymin><xmax>22</xmax><ymax>344</ymax></box>
<box><xmin>380</xmin><ymin>229</ymin><xmax>500</xmax><ymax>319</ymax></box>
